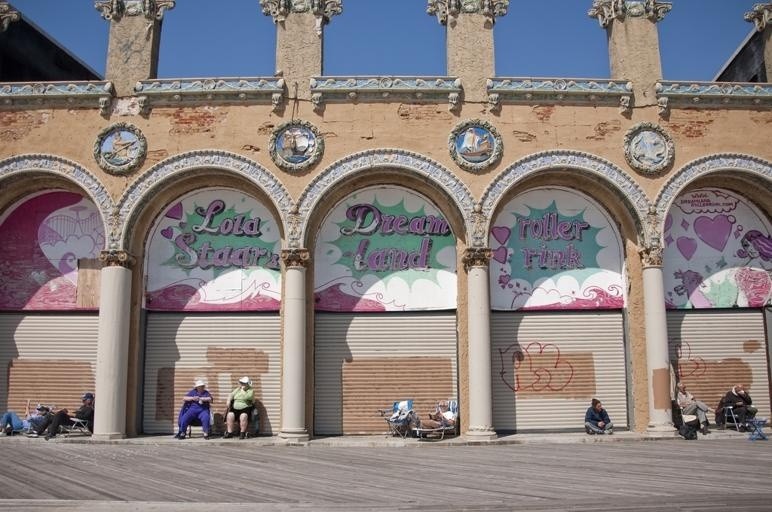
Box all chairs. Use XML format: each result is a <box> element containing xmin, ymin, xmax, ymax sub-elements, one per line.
<box><xmin>180</xmin><ymin>432</ymin><xmax>186</xmax><ymax>439</ymax></box>
<box><xmin>739</xmin><ymin>422</ymin><xmax>752</xmax><ymax>433</ymax></box>
<box><xmin>22</xmin><ymin>430</ymin><xmax>39</xmax><ymax>438</ymax></box>
<box><xmin>204</xmin><ymin>432</ymin><xmax>210</xmax><ymax>439</ymax></box>
<box><xmin>223</xmin><ymin>430</ymin><xmax>246</xmax><ymax>439</ymax></box>
<box><xmin>0</xmin><ymin>431</ymin><xmax>7</xmax><ymax>437</ymax></box>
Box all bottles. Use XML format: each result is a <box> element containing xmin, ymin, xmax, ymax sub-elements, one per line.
<box><xmin>592</xmin><ymin>398</ymin><xmax>600</xmax><ymax>408</ymax></box>
<box><xmin>239</xmin><ymin>376</ymin><xmax>249</xmax><ymax>384</ymax></box>
<box><xmin>193</xmin><ymin>379</ymin><xmax>206</xmax><ymax>390</ymax></box>
<box><xmin>80</xmin><ymin>392</ymin><xmax>94</xmax><ymax>402</ymax></box>
<box><xmin>36</xmin><ymin>404</ymin><xmax>50</xmax><ymax>412</ymax></box>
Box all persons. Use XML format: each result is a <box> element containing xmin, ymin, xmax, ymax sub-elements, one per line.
<box><xmin>676</xmin><ymin>382</ymin><xmax>724</xmax><ymax>435</ymax></box>
<box><xmin>0</xmin><ymin>398</ymin><xmax>50</xmax><ymax>436</ymax></box>
<box><xmin>223</xmin><ymin>376</ymin><xmax>256</xmax><ymax>439</ymax></box>
<box><xmin>23</xmin><ymin>392</ymin><xmax>95</xmax><ymax>440</ymax></box>
<box><xmin>407</xmin><ymin>400</ymin><xmax>457</xmax><ymax>428</ymax></box>
<box><xmin>175</xmin><ymin>378</ymin><xmax>214</xmax><ymax>440</ymax></box>
<box><xmin>584</xmin><ymin>398</ymin><xmax>613</xmax><ymax>434</ymax></box>
<box><xmin>715</xmin><ymin>383</ymin><xmax>758</xmax><ymax>432</ymax></box>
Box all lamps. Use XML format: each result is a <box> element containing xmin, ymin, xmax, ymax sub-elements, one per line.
<box><xmin>11</xmin><ymin>406</ymin><xmax>55</xmax><ymax>436</ymax></box>
<box><xmin>59</xmin><ymin>418</ymin><xmax>90</xmax><ymax>437</ymax></box>
<box><xmin>725</xmin><ymin>407</ymin><xmax>739</xmax><ymax>432</ymax></box>
<box><xmin>681</xmin><ymin>409</ymin><xmax>698</xmax><ymax>426</ymax></box>
<box><xmin>378</xmin><ymin>400</ymin><xmax>413</xmax><ymax>438</ymax></box>
<box><xmin>413</xmin><ymin>401</ymin><xmax>457</xmax><ymax>440</ymax></box>
<box><xmin>189</xmin><ymin>419</ymin><xmax>212</xmax><ymax>437</ymax></box>
